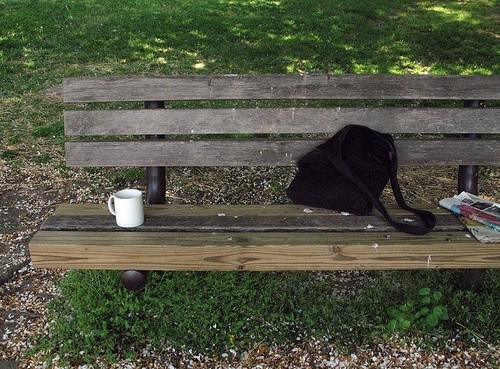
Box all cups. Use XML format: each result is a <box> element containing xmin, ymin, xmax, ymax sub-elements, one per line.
<box><xmin>108</xmin><ymin>189</ymin><xmax>145</xmax><ymax>227</ymax></box>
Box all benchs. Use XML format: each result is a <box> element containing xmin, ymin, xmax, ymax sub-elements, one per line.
<box><xmin>28</xmin><ymin>74</ymin><xmax>500</xmax><ymax>292</ymax></box>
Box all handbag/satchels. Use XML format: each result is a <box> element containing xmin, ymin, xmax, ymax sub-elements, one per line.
<box><xmin>283</xmin><ymin>121</ymin><xmax>438</xmax><ymax>235</ymax></box>
